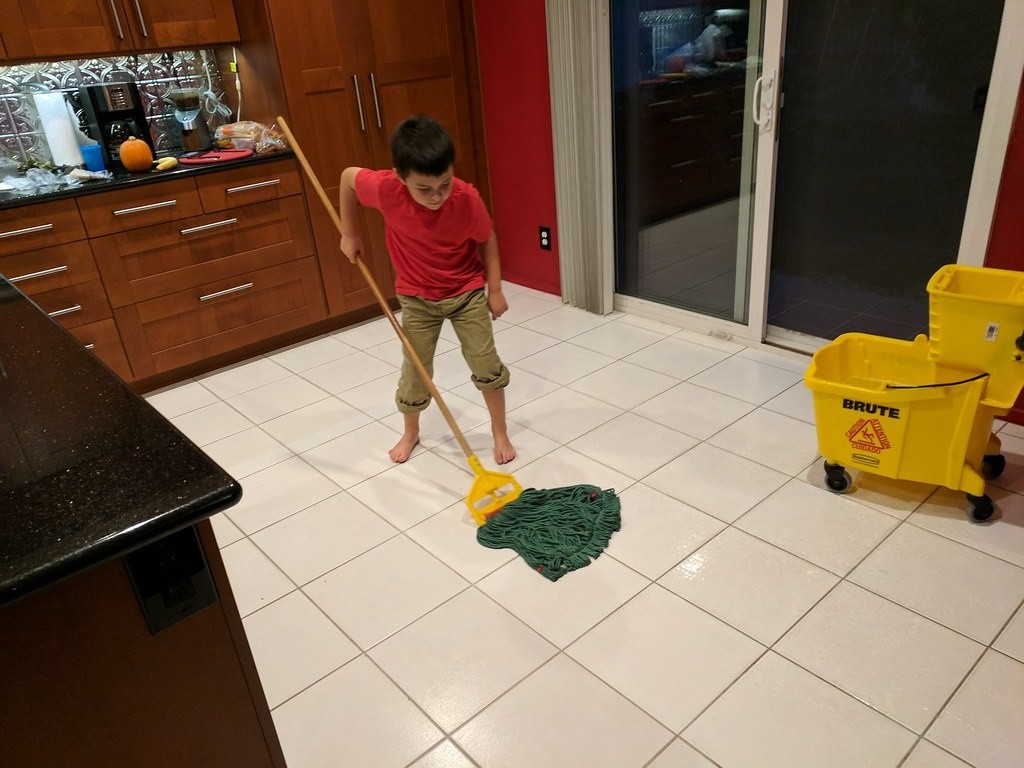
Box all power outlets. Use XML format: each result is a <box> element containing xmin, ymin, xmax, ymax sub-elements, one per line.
<box><xmin>539</xmin><ymin>225</ymin><xmax>552</xmax><ymax>250</ymax></box>
<box><xmin>121</xmin><ymin>526</ymin><xmax>218</xmax><ymax>635</ymax></box>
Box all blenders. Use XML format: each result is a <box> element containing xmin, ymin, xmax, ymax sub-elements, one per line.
<box><xmin>171</xmin><ymin>88</ymin><xmax>210</xmax><ymax>152</ymax></box>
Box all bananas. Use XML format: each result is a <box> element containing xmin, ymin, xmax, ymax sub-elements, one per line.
<box><xmin>659</xmin><ymin>73</ymin><xmax>687</xmax><ymax>77</ymax></box>
<box><xmin>152</xmin><ymin>157</ymin><xmax>178</xmax><ymax>170</ymax></box>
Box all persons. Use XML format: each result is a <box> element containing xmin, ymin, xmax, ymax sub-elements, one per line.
<box><xmin>339</xmin><ymin>113</ymin><xmax>518</xmax><ymax>466</ymax></box>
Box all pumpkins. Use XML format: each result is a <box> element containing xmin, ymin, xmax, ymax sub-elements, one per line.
<box><xmin>667</xmin><ymin>58</ymin><xmax>684</xmax><ymax>72</ymax></box>
<box><xmin>119</xmin><ymin>135</ymin><xmax>153</xmax><ymax>170</ymax></box>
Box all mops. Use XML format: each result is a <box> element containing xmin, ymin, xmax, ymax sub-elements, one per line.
<box><xmin>272</xmin><ymin>113</ymin><xmax>622</xmax><ymax>584</ymax></box>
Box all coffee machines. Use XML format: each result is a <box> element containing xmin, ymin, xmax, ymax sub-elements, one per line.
<box><xmin>79</xmin><ymin>82</ymin><xmax>157</xmax><ymax>165</ymax></box>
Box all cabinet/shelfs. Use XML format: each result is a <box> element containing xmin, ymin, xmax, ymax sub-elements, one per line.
<box><xmin>75</xmin><ymin>159</ymin><xmax>330</xmax><ymax>382</ymax></box>
<box><xmin>0</xmin><ymin>0</ymin><xmax>240</xmax><ymax>60</ymax></box>
<box><xmin>267</xmin><ymin>0</ymin><xmax>478</xmax><ymax>319</ymax></box>
<box><xmin>634</xmin><ymin>79</ymin><xmax>720</xmax><ymax>218</ymax></box>
<box><xmin>721</xmin><ymin>74</ymin><xmax>745</xmax><ymax>195</ymax></box>
<box><xmin>0</xmin><ymin>198</ymin><xmax>135</xmax><ymax>385</ymax></box>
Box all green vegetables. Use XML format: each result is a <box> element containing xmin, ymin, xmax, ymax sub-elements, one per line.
<box><xmin>17</xmin><ymin>158</ymin><xmax>55</xmax><ymax>173</ymax></box>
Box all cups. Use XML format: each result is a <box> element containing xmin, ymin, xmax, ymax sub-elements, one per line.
<box><xmin>81</xmin><ymin>145</ymin><xmax>105</xmax><ymax>172</ymax></box>
